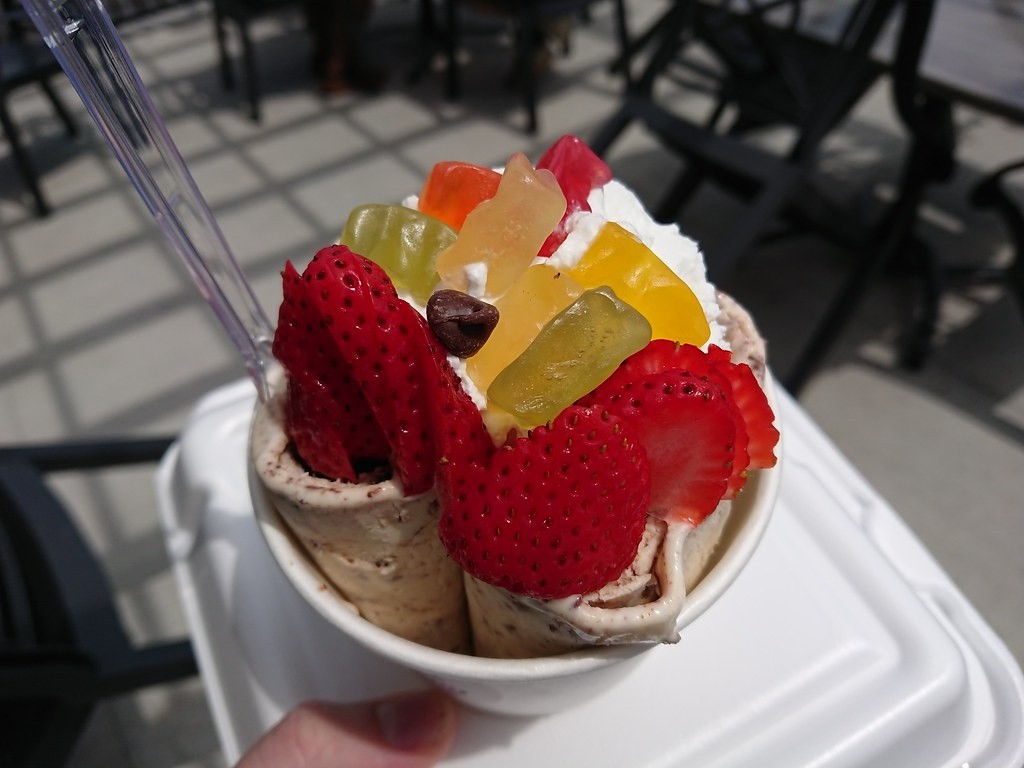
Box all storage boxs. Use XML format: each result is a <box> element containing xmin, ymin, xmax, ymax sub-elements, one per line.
<box><xmin>157</xmin><ymin>365</ymin><xmax>1024</xmax><ymax>768</ymax></box>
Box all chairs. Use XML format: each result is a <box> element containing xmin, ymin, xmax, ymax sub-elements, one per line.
<box><xmin>0</xmin><ymin>0</ymin><xmax>147</xmax><ymax>218</ymax></box>
<box><xmin>585</xmin><ymin>0</ymin><xmax>960</xmax><ymax>398</ymax></box>
<box><xmin>205</xmin><ymin>0</ymin><xmax>577</xmax><ymax>129</ymax></box>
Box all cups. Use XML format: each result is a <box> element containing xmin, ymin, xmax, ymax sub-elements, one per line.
<box><xmin>247</xmin><ymin>394</ymin><xmax>781</xmax><ymax>718</ymax></box>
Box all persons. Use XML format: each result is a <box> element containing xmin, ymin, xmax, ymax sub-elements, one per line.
<box><xmin>233</xmin><ymin>683</ymin><xmax>459</xmax><ymax>768</ymax></box>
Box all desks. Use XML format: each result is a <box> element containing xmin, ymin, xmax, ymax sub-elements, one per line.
<box><xmin>730</xmin><ymin>0</ymin><xmax>1024</xmax><ymax>127</ymax></box>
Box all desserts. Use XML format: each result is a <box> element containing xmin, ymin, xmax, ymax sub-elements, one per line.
<box><xmin>250</xmin><ymin>134</ymin><xmax>780</xmax><ymax>663</ymax></box>
